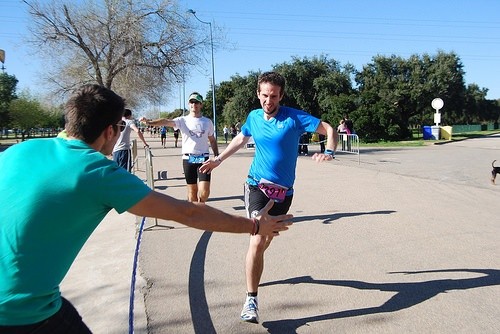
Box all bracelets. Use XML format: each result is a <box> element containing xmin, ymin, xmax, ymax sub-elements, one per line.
<box><xmin>250</xmin><ymin>217</ymin><xmax>261</xmax><ymax>237</ymax></box>
<box><xmin>215</xmin><ymin>155</ymin><xmax>222</xmax><ymax>163</ymax></box>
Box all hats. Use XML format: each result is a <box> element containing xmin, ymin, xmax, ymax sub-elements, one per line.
<box><xmin>188</xmin><ymin>94</ymin><xmax>203</xmax><ymax>103</ymax></box>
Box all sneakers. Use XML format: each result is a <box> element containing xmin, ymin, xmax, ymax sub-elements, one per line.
<box><xmin>241</xmin><ymin>296</ymin><xmax>260</xmax><ymax>325</ymax></box>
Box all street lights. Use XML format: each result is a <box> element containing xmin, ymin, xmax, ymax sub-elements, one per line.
<box><xmin>188</xmin><ymin>8</ymin><xmax>218</xmax><ymax>143</ymax></box>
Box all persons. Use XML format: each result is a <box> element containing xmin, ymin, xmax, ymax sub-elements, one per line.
<box><xmin>198</xmin><ymin>71</ymin><xmax>339</xmax><ymax>324</ymax></box>
<box><xmin>223</xmin><ymin>119</ymin><xmax>241</xmax><ymax>144</ymax></box>
<box><xmin>318</xmin><ymin>133</ymin><xmax>326</xmax><ymax>154</ymax></box>
<box><xmin>140</xmin><ymin>123</ymin><xmax>179</xmax><ymax>149</ymax></box>
<box><xmin>0</xmin><ymin>84</ymin><xmax>295</xmax><ymax>334</ymax></box>
<box><xmin>337</xmin><ymin>115</ymin><xmax>355</xmax><ymax>152</ymax></box>
<box><xmin>138</xmin><ymin>92</ymin><xmax>220</xmax><ymax>206</ymax></box>
<box><xmin>112</xmin><ymin>108</ymin><xmax>150</xmax><ymax>175</ymax></box>
<box><xmin>297</xmin><ymin>131</ymin><xmax>312</xmax><ymax>156</ymax></box>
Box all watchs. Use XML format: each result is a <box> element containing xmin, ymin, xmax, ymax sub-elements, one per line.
<box><xmin>324</xmin><ymin>149</ymin><xmax>335</xmax><ymax>158</ymax></box>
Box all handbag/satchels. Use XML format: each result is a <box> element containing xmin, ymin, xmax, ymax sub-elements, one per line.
<box><xmin>257</xmin><ymin>180</ymin><xmax>288</xmax><ymax>203</ymax></box>
<box><xmin>185</xmin><ymin>153</ymin><xmax>208</xmax><ymax>163</ymax></box>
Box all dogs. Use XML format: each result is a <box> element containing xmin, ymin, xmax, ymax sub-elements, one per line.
<box><xmin>490</xmin><ymin>159</ymin><xmax>500</xmax><ymax>184</ymax></box>
